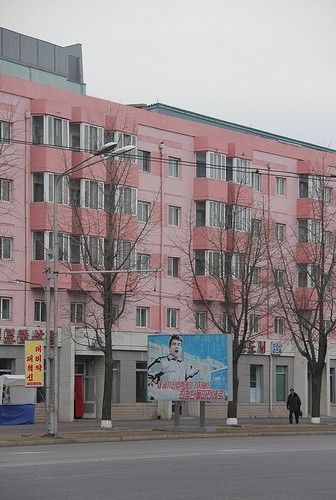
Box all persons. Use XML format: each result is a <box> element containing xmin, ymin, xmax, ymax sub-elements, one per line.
<box><xmin>287</xmin><ymin>389</ymin><xmax>301</xmax><ymax>424</ymax></box>
<box><xmin>147</xmin><ymin>335</ymin><xmax>199</xmax><ymax>389</ymax></box>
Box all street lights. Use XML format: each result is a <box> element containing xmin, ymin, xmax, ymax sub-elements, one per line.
<box><xmin>7</xmin><ymin>141</ymin><xmax>138</xmax><ymax>437</ymax></box>
